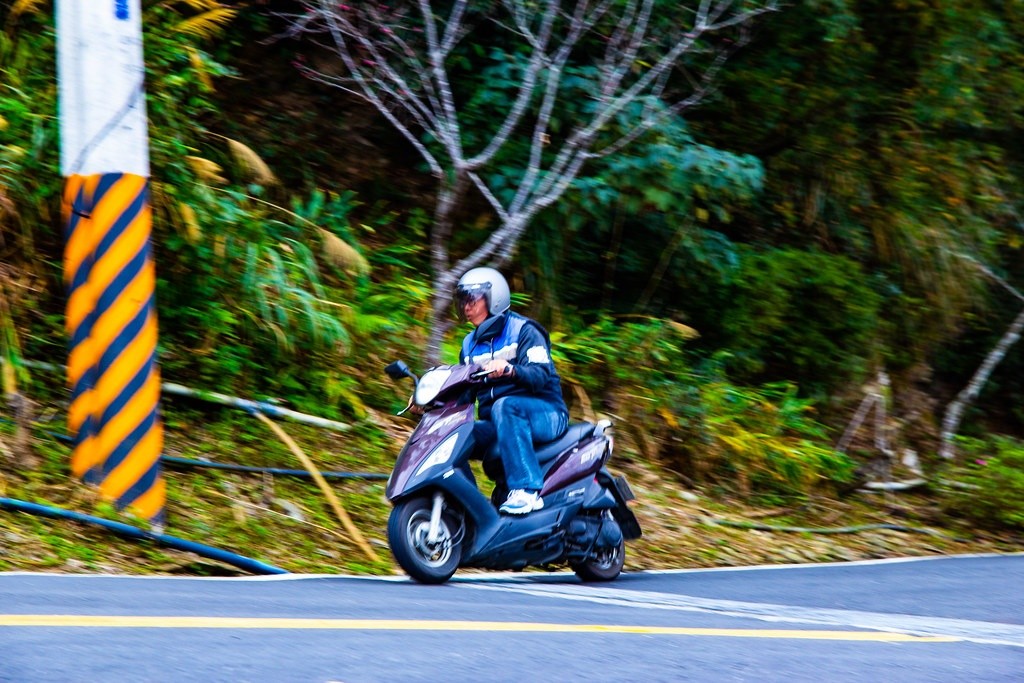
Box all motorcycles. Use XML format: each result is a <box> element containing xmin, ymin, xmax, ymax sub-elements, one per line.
<box><xmin>383</xmin><ymin>330</ymin><xmax>644</xmax><ymax>583</ymax></box>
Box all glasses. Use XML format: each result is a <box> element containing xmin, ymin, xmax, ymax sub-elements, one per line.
<box><xmin>459</xmin><ymin>297</ymin><xmax>485</xmax><ymax>308</ymax></box>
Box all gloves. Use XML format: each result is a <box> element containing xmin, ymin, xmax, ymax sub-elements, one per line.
<box><xmin>408</xmin><ymin>395</ymin><xmax>427</xmax><ymax>415</ymax></box>
<box><xmin>483</xmin><ymin>357</ymin><xmax>513</xmax><ymax>377</ymax></box>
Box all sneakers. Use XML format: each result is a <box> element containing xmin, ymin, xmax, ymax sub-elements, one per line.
<box><xmin>500</xmin><ymin>489</ymin><xmax>545</xmax><ymax>515</ymax></box>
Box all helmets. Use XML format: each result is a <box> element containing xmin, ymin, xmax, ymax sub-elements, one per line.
<box><xmin>457</xmin><ymin>267</ymin><xmax>511</xmax><ymax>315</ymax></box>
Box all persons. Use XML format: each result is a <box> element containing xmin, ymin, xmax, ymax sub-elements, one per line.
<box><xmin>408</xmin><ymin>266</ymin><xmax>570</xmax><ymax>514</ymax></box>
